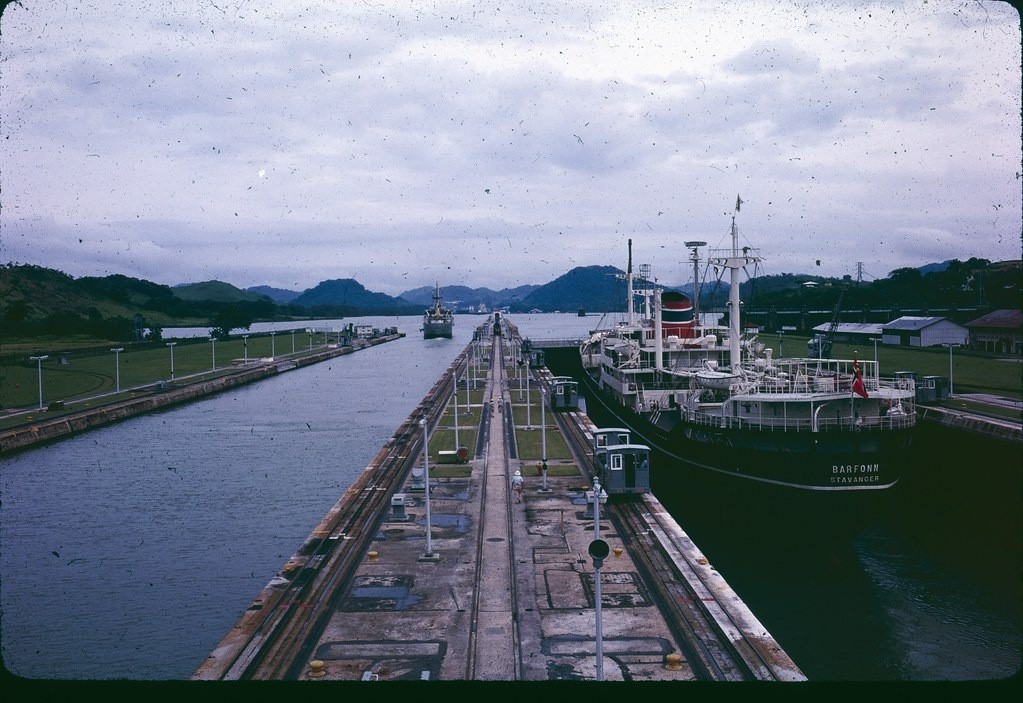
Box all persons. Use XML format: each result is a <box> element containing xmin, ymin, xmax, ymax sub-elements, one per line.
<box><xmin>880</xmin><ymin>399</ymin><xmax>896</xmax><ymax>416</ymax></box>
<box><xmin>511</xmin><ymin>470</ymin><xmax>524</xmax><ymax>504</ymax></box>
<box><xmin>854</xmin><ymin>404</ymin><xmax>861</xmax><ymax>418</ymax></box>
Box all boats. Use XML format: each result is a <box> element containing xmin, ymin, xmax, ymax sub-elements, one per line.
<box><xmin>573</xmin><ymin>192</ymin><xmax>919</xmax><ymax>521</ymax></box>
<box><xmin>422</xmin><ymin>280</ymin><xmax>456</xmax><ymax>340</ymax></box>
<box><xmin>693</xmin><ymin>358</ymin><xmax>745</xmax><ymax>391</ymax></box>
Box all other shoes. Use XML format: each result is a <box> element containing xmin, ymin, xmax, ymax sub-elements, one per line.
<box><xmin>516</xmin><ymin>500</ymin><xmax>521</xmax><ymax>504</ymax></box>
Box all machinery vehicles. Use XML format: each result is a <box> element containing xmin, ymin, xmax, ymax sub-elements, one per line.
<box><xmin>805</xmin><ymin>282</ymin><xmax>850</xmax><ymax>366</ymax></box>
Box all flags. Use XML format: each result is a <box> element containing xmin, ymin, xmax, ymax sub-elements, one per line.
<box><xmin>852</xmin><ymin>356</ymin><xmax>869</xmax><ymax>399</ymax></box>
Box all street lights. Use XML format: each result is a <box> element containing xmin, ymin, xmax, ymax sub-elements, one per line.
<box><xmin>776</xmin><ymin>330</ymin><xmax>784</xmax><ymax>358</ymax></box>
<box><xmin>585</xmin><ymin>476</ymin><xmax>608</xmax><ymax>679</ymax></box>
<box><xmin>465</xmin><ymin>354</ymin><xmax>470</xmax><ymax>413</ymax></box>
<box><xmin>451</xmin><ymin>371</ymin><xmax>459</xmax><ymax>451</ymax></box>
<box><xmin>209</xmin><ymin>338</ymin><xmax>218</xmax><ymax>371</ymax></box>
<box><xmin>242</xmin><ymin>334</ymin><xmax>249</xmax><ymax>364</ymax></box>
<box><xmin>542</xmin><ymin>388</ymin><xmax>547</xmax><ymax>490</ymax></box>
<box><xmin>270</xmin><ymin>332</ymin><xmax>275</xmax><ymax>358</ymax></box>
<box><xmin>869</xmin><ymin>337</ymin><xmax>882</xmax><ymax>379</ymax></box>
<box><xmin>291</xmin><ymin>329</ymin><xmax>295</xmax><ymax>354</ymax></box>
<box><xmin>166</xmin><ymin>342</ymin><xmax>177</xmax><ymax>380</ymax></box>
<box><xmin>519</xmin><ymin>348</ymin><xmax>522</xmax><ymax>400</ymax></box>
<box><xmin>527</xmin><ymin>361</ymin><xmax>531</xmax><ymax>429</ymax></box>
<box><xmin>418</xmin><ymin>414</ymin><xmax>434</xmax><ymax>557</ymax></box>
<box><xmin>111</xmin><ymin>348</ymin><xmax>124</xmax><ymax>392</ymax></box>
<box><xmin>815</xmin><ymin>334</ymin><xmax>825</xmax><ymax>370</ymax></box>
<box><xmin>941</xmin><ymin>343</ymin><xmax>960</xmax><ymax>398</ymax></box>
<box><xmin>30</xmin><ymin>355</ymin><xmax>49</xmax><ymax>408</ymax></box>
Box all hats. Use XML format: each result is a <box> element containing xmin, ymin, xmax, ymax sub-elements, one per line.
<box><xmin>515</xmin><ymin>471</ymin><xmax>521</xmax><ymax>475</ymax></box>
